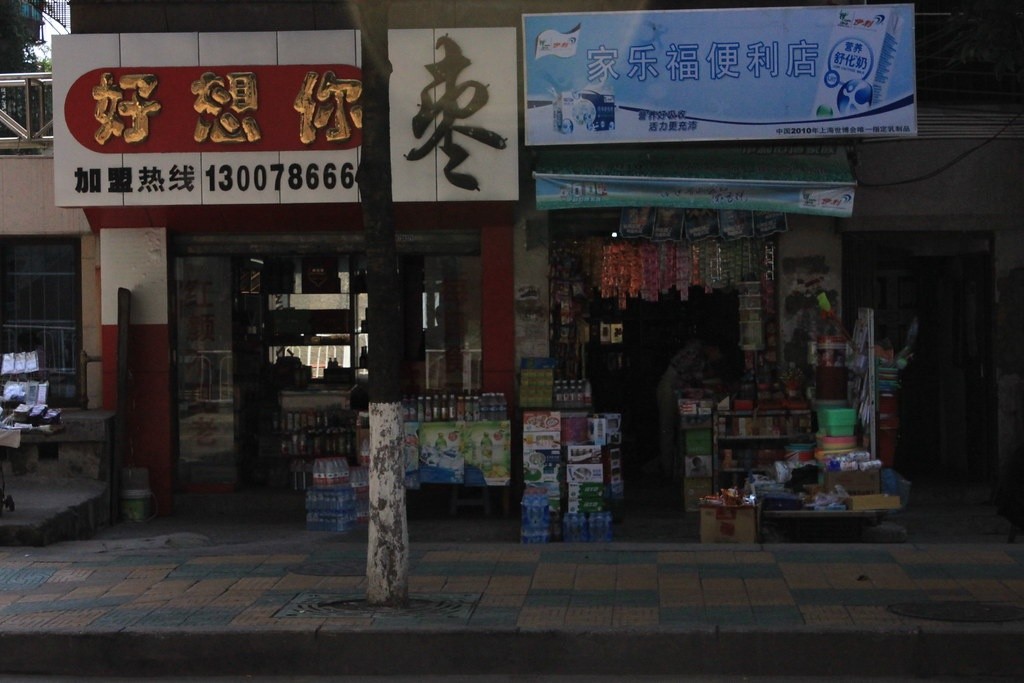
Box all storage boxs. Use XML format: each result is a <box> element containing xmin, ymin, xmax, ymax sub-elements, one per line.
<box><xmin>685</xmin><ymin>429</ymin><xmax>713</xmax><ymax>510</ymax></box>
<box><xmin>701</xmin><ymin>502</ymin><xmax>762</xmax><ymax>545</ymax></box>
<box><xmin>826</xmin><ymin>467</ymin><xmax>901</xmax><ymax>509</ymax></box>
<box><xmin>524</xmin><ymin>409</ymin><xmax>621</xmax><ymax>515</ymax></box>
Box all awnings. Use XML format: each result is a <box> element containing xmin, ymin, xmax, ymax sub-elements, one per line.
<box><xmin>533</xmin><ymin>142</ymin><xmax>860</xmax><ymax>216</ymax></box>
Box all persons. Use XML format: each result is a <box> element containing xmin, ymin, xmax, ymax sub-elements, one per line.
<box><xmin>19</xmin><ymin>332</ymin><xmax>50</xmax><ymax>381</ymax></box>
<box><xmin>658</xmin><ymin>335</ymin><xmax>727</xmax><ymax>450</ymax></box>
<box><xmin>688</xmin><ymin>458</ymin><xmax>709</xmax><ymax>478</ymax></box>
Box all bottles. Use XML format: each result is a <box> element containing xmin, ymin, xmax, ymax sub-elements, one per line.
<box><xmin>361</xmin><ymin>448</ymin><xmax>370</xmax><ymax>465</ymax></box>
<box><xmin>305</xmin><ymin>511</ymin><xmax>355</xmax><ymax>533</ymax></box>
<box><xmin>520</xmin><ymin>528</ymin><xmax>550</xmax><ymax>544</ymax></box>
<box><xmin>401</xmin><ymin>392</ymin><xmax>507</xmax><ymax>421</ymax></box>
<box><xmin>581</xmin><ymin>483</ymin><xmax>604</xmax><ymax>511</ymax></box>
<box><xmin>282</xmin><ymin>428</ymin><xmax>353</xmax><ymax>456</ymax></box>
<box><xmin>285</xmin><ymin>409</ymin><xmax>328</xmax><ymax>434</ymax></box>
<box><xmin>435</xmin><ymin>432</ymin><xmax>447</xmax><ymax>453</ymax></box>
<box><xmin>562</xmin><ymin>511</ymin><xmax>612</xmax><ymax>542</ymax></box>
<box><xmin>520</xmin><ymin>491</ymin><xmax>550</xmax><ymax>528</ymax></box>
<box><xmin>304</xmin><ymin>486</ymin><xmax>369</xmax><ymax>511</ymax></box>
<box><xmin>353</xmin><ymin>509</ymin><xmax>370</xmax><ymax>525</ymax></box>
<box><xmin>349</xmin><ymin>466</ymin><xmax>369</xmax><ymax>493</ymax></box>
<box><xmin>481</xmin><ymin>432</ymin><xmax>493</xmax><ymax>471</ymax></box>
<box><xmin>312</xmin><ymin>458</ymin><xmax>350</xmax><ymax>485</ymax></box>
<box><xmin>554</xmin><ymin>379</ymin><xmax>592</xmax><ymax>410</ymax></box>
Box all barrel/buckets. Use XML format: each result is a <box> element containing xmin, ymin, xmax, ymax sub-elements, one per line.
<box><xmin>816</xmin><ymin>366</ymin><xmax>847</xmax><ymax>399</ymax></box>
<box><xmin>816</xmin><ymin>335</ymin><xmax>847</xmax><ymax>366</ymax></box>
